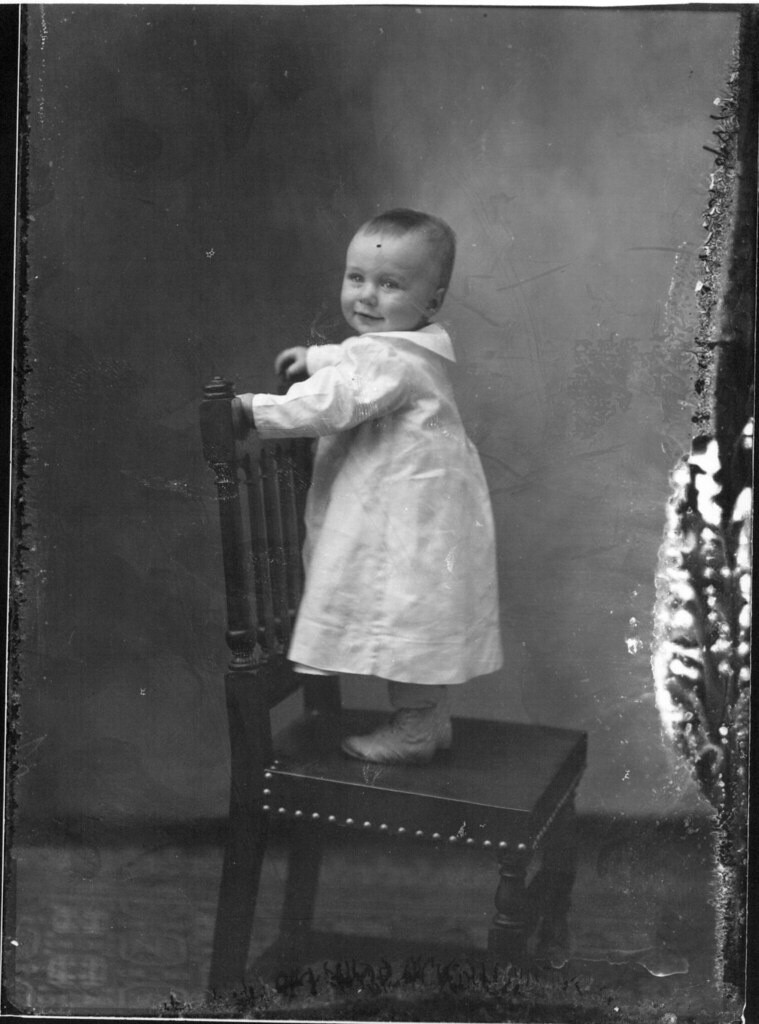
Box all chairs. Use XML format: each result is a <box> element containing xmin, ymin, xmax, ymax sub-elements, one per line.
<box><xmin>200</xmin><ymin>376</ymin><xmax>587</xmax><ymax>1016</ymax></box>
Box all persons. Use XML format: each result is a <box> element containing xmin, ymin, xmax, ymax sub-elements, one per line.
<box><xmin>234</xmin><ymin>208</ymin><xmax>502</xmax><ymax>764</ymax></box>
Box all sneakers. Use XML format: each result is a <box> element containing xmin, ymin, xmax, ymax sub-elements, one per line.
<box><xmin>434</xmin><ymin>721</ymin><xmax>453</xmax><ymax>750</ymax></box>
<box><xmin>340</xmin><ymin>720</ymin><xmax>435</xmax><ymax>765</ymax></box>
